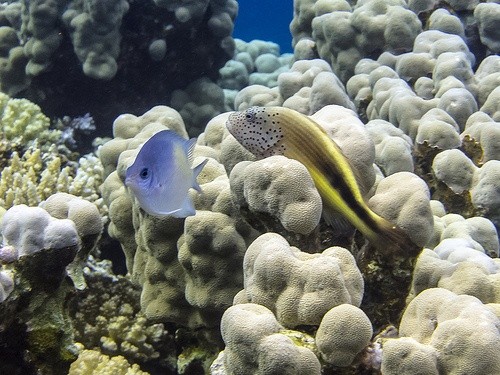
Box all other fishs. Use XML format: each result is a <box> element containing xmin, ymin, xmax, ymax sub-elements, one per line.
<box><xmin>226</xmin><ymin>107</ymin><xmax>422</xmax><ymax>261</ymax></box>
<box><xmin>126</xmin><ymin>130</ymin><xmax>208</xmax><ymax>220</ymax></box>
